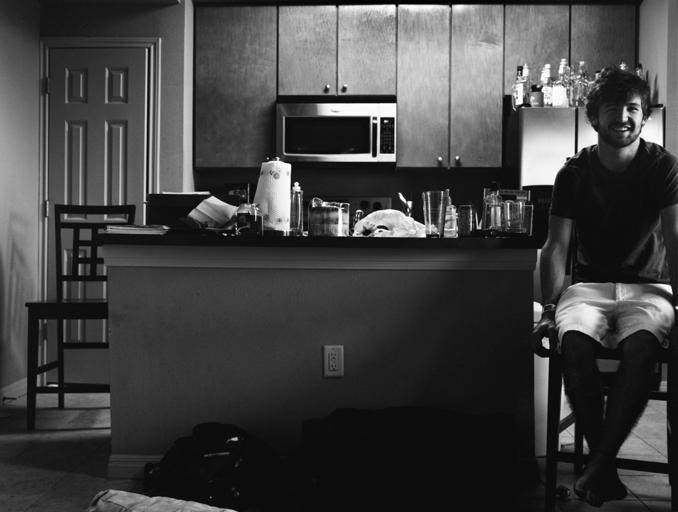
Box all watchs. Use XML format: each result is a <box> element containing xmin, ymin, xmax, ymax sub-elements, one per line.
<box><xmin>540</xmin><ymin>303</ymin><xmax>557</xmax><ymax>316</ymax></box>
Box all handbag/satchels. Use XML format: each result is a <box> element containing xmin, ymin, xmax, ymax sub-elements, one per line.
<box><xmin>144</xmin><ymin>422</ymin><xmax>259</xmax><ymax>511</ymax></box>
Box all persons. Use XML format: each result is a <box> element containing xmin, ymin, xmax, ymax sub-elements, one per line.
<box><xmin>534</xmin><ymin>68</ymin><xmax>678</xmax><ymax>510</ymax></box>
